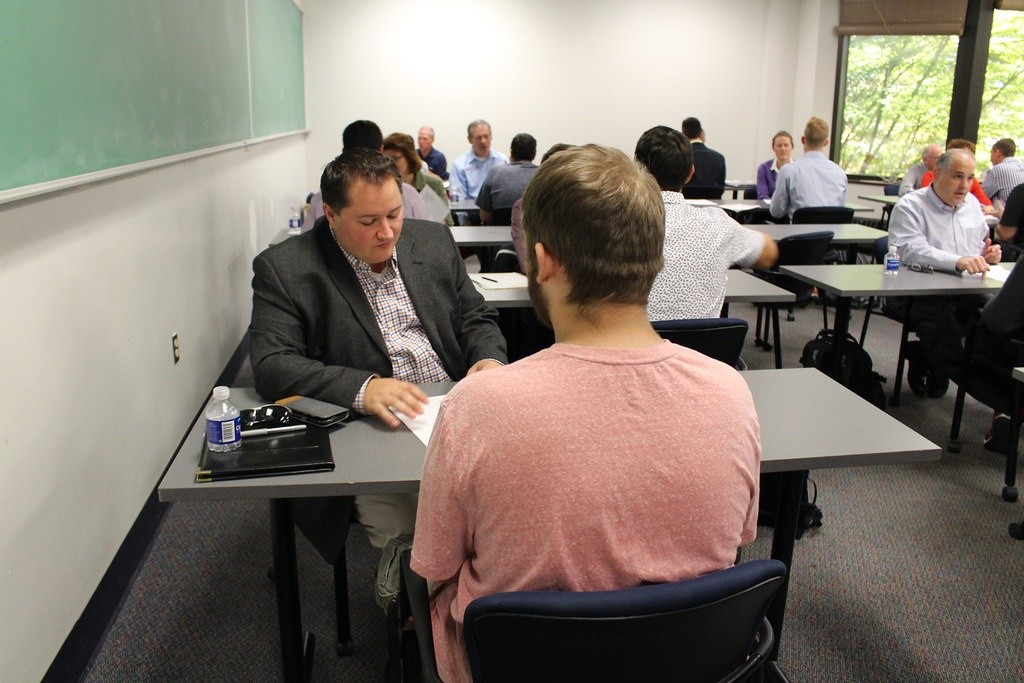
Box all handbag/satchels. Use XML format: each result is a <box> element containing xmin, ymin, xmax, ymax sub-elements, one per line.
<box><xmin>796</xmin><ymin>327</ymin><xmax>887</xmax><ymax>410</ymax></box>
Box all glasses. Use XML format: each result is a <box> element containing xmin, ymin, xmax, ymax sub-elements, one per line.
<box><xmin>240</xmin><ymin>404</ymin><xmax>292</xmax><ymax>429</ymax></box>
<box><xmin>389</xmin><ymin>153</ymin><xmax>404</xmax><ymax>165</ymax></box>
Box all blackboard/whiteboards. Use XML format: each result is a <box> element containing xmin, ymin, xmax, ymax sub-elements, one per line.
<box><xmin>0</xmin><ymin>0</ymin><xmax>312</xmax><ymax>203</ymax></box>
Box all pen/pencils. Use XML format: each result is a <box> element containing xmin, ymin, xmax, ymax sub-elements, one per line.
<box><xmin>472</xmin><ymin>280</ymin><xmax>488</xmax><ymax>289</ymax></box>
<box><xmin>482</xmin><ymin>277</ymin><xmax>497</xmax><ymax>283</ymax></box>
<box><xmin>241</xmin><ymin>424</ymin><xmax>307</xmax><ymax>436</ymax></box>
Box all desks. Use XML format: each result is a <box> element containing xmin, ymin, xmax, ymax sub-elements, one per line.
<box><xmin>460</xmin><ymin>270</ymin><xmax>798</xmax><ymax>373</ymax></box>
<box><xmin>721</xmin><ymin>271</ymin><xmax>795</xmax><ymax>366</ymax></box>
<box><xmin>447</xmin><ymin>197</ymin><xmax>481</xmax><ymax>212</ymax></box>
<box><xmin>685</xmin><ymin>196</ymin><xmax>874</xmax><ymax>224</ymax></box>
<box><xmin>777</xmin><ymin>261</ymin><xmax>1016</xmax><ymax>452</ymax></box>
<box><xmin>724</xmin><ymin>181</ymin><xmax>759</xmax><ymax>189</ymax></box>
<box><xmin>739</xmin><ymin>221</ymin><xmax>889</xmax><ymax>346</ymax></box>
<box><xmin>857</xmin><ymin>190</ymin><xmax>912</xmax><ymax>226</ymax></box>
<box><xmin>443</xmin><ymin>224</ymin><xmax>518</xmax><ymax>246</ymax></box>
<box><xmin>158</xmin><ymin>366</ymin><xmax>943</xmax><ymax>683</ymax></box>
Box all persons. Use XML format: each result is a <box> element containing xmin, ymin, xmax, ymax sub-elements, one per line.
<box><xmin>899</xmin><ymin>138</ymin><xmax>1024</xmax><ymax>262</ymax></box>
<box><xmin>768</xmin><ymin>116</ymin><xmax>847</xmax><ymax>224</ymax></box>
<box><xmin>681</xmin><ymin>117</ymin><xmax>726</xmax><ymax>199</ymax></box>
<box><xmin>303</xmin><ymin>120</ymin><xmax>428</xmax><ymax>234</ymax></box>
<box><xmin>247</xmin><ymin>151</ymin><xmax>509</xmax><ymax>619</ymax></box>
<box><xmin>978</xmin><ymin>253</ymin><xmax>1024</xmax><ymax>456</ymax></box>
<box><xmin>882</xmin><ymin>148</ymin><xmax>1002</xmax><ymax>397</ymax></box>
<box><xmin>382</xmin><ymin>120</ymin><xmax>577</xmax><ymax>274</ymax></box>
<box><xmin>410</xmin><ymin>142</ymin><xmax>762</xmax><ymax>682</ymax></box>
<box><xmin>757</xmin><ymin>130</ymin><xmax>795</xmax><ymax>199</ymax></box>
<box><xmin>632</xmin><ymin>126</ymin><xmax>778</xmax><ymax>322</ymax></box>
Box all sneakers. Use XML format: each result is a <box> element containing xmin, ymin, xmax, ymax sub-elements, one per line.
<box><xmin>983</xmin><ymin>410</ymin><xmax>1011</xmax><ymax>449</ymax></box>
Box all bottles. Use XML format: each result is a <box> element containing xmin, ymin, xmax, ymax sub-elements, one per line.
<box><xmin>884</xmin><ymin>246</ymin><xmax>901</xmax><ymax>275</ymax></box>
<box><xmin>906</xmin><ymin>185</ymin><xmax>914</xmax><ymax>193</ymax></box>
<box><xmin>288</xmin><ymin>208</ymin><xmax>302</xmax><ymax>236</ymax></box>
<box><xmin>449</xmin><ymin>187</ymin><xmax>459</xmax><ymax>209</ymax></box>
<box><xmin>205</xmin><ymin>386</ymin><xmax>242</xmax><ymax>453</ymax></box>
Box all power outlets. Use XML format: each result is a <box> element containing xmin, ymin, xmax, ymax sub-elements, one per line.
<box><xmin>173</xmin><ymin>334</ymin><xmax>180</xmax><ymax>363</ymax></box>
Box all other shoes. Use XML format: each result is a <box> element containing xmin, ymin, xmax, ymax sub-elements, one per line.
<box><xmin>925</xmin><ymin>343</ymin><xmax>949</xmax><ymax>397</ymax></box>
<box><xmin>402</xmin><ymin>615</ymin><xmax>416</xmax><ymax>629</ymax></box>
<box><xmin>813</xmin><ymin>294</ymin><xmax>835</xmax><ymax>306</ymax></box>
<box><xmin>905</xmin><ymin>339</ymin><xmax>928</xmax><ymax>400</ymax></box>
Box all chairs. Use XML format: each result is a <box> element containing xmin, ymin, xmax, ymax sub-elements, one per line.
<box><xmin>272</xmin><ymin>189</ymin><xmax>1024</xmax><ymax>683</ymax></box>
